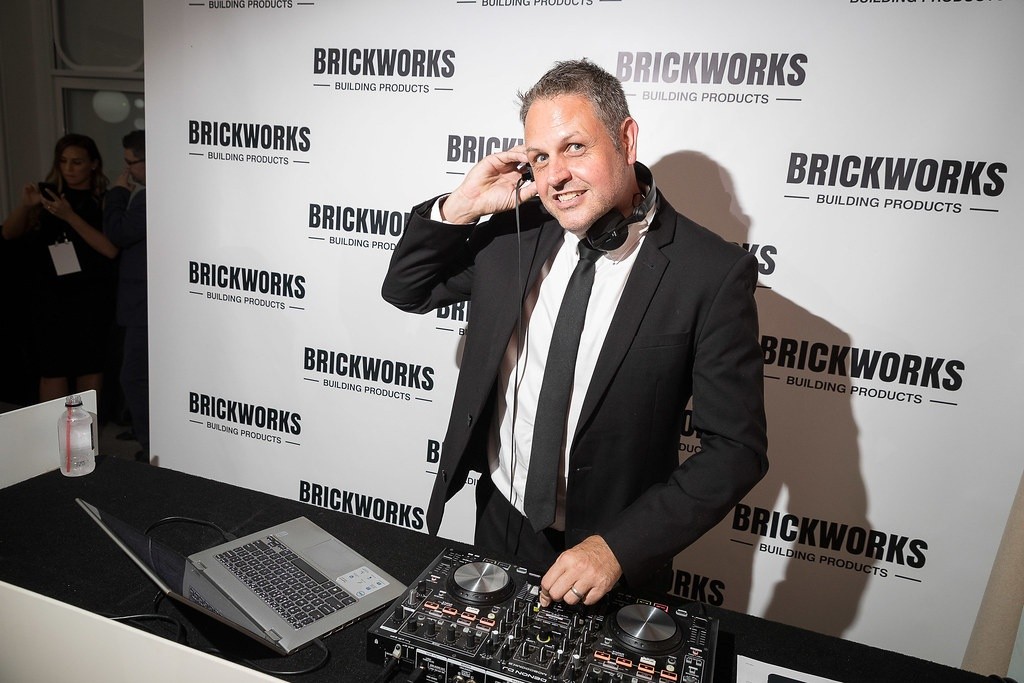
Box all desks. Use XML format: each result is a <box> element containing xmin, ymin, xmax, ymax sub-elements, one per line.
<box><xmin>0</xmin><ymin>455</ymin><xmax>1018</xmax><ymax>683</ymax></box>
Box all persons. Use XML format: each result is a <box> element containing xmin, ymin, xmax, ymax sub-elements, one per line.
<box><xmin>104</xmin><ymin>130</ymin><xmax>149</xmax><ymax>462</ymax></box>
<box><xmin>381</xmin><ymin>63</ymin><xmax>769</xmax><ymax>607</ymax></box>
<box><xmin>2</xmin><ymin>133</ymin><xmax>121</xmax><ymax>406</ymax></box>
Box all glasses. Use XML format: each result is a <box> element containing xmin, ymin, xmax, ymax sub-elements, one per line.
<box><xmin>123</xmin><ymin>157</ymin><xmax>146</xmax><ymax>166</ymax></box>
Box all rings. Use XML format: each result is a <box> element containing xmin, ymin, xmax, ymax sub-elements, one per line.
<box><xmin>571</xmin><ymin>586</ymin><xmax>582</xmax><ymax>598</ymax></box>
<box><xmin>47</xmin><ymin>205</ymin><xmax>52</xmax><ymax>210</ymax></box>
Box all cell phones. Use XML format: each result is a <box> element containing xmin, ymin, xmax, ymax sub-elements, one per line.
<box><xmin>38</xmin><ymin>181</ymin><xmax>62</xmax><ymax>202</ymax></box>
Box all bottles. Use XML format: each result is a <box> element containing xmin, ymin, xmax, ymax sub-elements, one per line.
<box><xmin>56</xmin><ymin>394</ymin><xmax>96</xmax><ymax>477</ymax></box>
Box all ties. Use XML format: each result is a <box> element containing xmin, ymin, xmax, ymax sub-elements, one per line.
<box><xmin>524</xmin><ymin>238</ymin><xmax>607</xmax><ymax>534</ymax></box>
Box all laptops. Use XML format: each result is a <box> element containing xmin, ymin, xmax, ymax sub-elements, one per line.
<box><xmin>73</xmin><ymin>497</ymin><xmax>412</xmax><ymax>656</ymax></box>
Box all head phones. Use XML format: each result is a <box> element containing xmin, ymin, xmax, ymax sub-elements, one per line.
<box><xmin>526</xmin><ymin>161</ymin><xmax>657</xmax><ymax>251</ymax></box>
